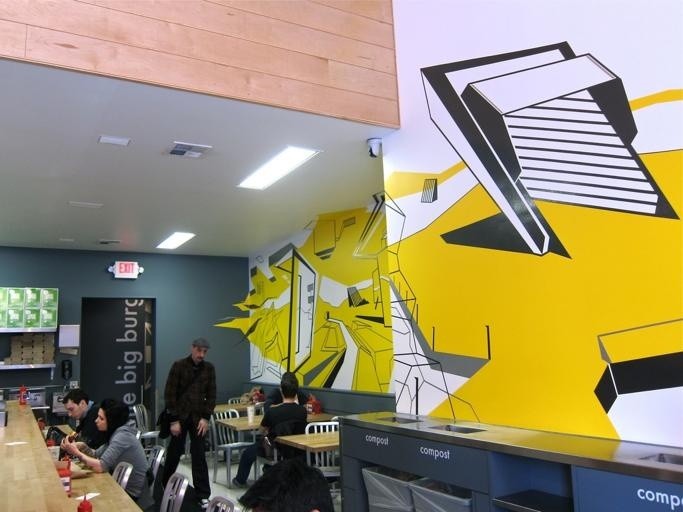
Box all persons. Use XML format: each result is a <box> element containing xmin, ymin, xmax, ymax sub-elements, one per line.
<box><xmin>62</xmin><ymin>387</ymin><xmax>109</xmax><ymax>450</ymax></box>
<box><xmin>60</xmin><ymin>398</ymin><xmax>149</xmax><ymax>503</ymax></box>
<box><xmin>236</xmin><ymin>457</ymin><xmax>335</xmax><ymax>512</ymax></box>
<box><xmin>263</xmin><ymin>389</ymin><xmax>309</xmax><ymax>414</ymax></box>
<box><xmin>162</xmin><ymin>335</ymin><xmax>217</xmax><ymax>509</ymax></box>
<box><xmin>231</xmin><ymin>371</ymin><xmax>307</xmax><ymax>489</ymax></box>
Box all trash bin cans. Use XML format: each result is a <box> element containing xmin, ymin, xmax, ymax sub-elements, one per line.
<box><xmin>491</xmin><ymin>489</ymin><xmax>574</xmax><ymax>512</ymax></box>
<box><xmin>361</xmin><ymin>466</ymin><xmax>425</xmax><ymax>512</ymax></box>
<box><xmin>408</xmin><ymin>477</ymin><xmax>472</xmax><ymax>512</ymax></box>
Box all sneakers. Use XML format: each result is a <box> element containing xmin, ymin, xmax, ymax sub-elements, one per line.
<box><xmin>232</xmin><ymin>476</ymin><xmax>248</xmax><ymax>488</ymax></box>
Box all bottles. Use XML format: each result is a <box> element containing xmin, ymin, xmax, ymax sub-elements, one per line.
<box><xmin>19</xmin><ymin>383</ymin><xmax>93</xmax><ymax>512</ymax></box>
<box><xmin>314</xmin><ymin>396</ymin><xmax>322</xmax><ymax>416</ymax></box>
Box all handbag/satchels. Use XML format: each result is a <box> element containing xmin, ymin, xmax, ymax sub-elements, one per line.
<box><xmin>156</xmin><ymin>408</ymin><xmax>173</xmax><ymax>439</ymax></box>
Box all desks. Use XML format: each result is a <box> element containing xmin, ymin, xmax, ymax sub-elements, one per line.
<box><xmin>39</xmin><ymin>422</ymin><xmax>146</xmax><ymax>512</ymax></box>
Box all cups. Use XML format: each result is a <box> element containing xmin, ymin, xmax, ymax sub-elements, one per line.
<box><xmin>246</xmin><ymin>406</ymin><xmax>255</xmax><ymax>423</ymax></box>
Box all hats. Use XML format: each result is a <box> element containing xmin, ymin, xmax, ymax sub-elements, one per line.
<box><xmin>191</xmin><ymin>337</ymin><xmax>211</xmax><ymax>348</ymax></box>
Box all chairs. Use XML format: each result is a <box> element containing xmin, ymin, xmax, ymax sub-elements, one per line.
<box><xmin>97</xmin><ymin>397</ymin><xmax>343</xmax><ymax>512</ymax></box>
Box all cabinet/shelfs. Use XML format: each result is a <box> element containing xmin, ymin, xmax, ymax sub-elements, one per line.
<box><xmin>0</xmin><ymin>326</ymin><xmax>58</xmax><ymax>380</ymax></box>
